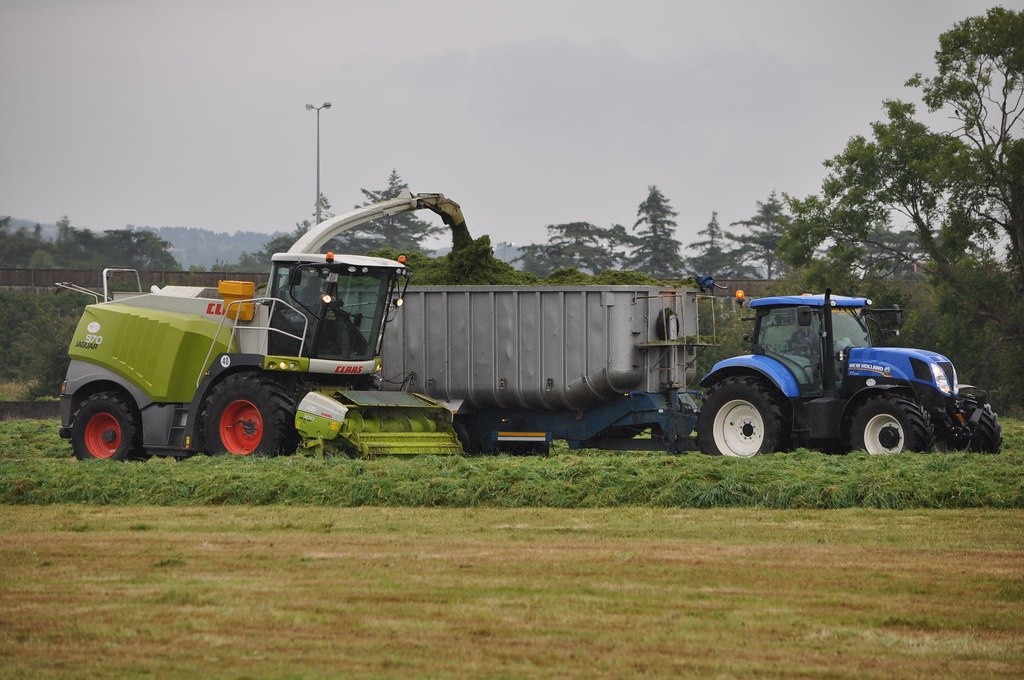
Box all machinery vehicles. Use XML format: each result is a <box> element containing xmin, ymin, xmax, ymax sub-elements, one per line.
<box><xmin>329</xmin><ymin>268</ymin><xmax>1002</xmax><ymax>460</ymax></box>
<box><xmin>51</xmin><ymin>190</ymin><xmax>464</xmax><ymax>463</ymax></box>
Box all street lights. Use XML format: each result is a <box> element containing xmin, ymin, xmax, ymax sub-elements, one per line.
<box><xmin>300</xmin><ymin>100</ymin><xmax>332</xmax><ymax>224</ymax></box>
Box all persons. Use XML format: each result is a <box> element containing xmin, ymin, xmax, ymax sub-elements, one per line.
<box><xmin>785</xmin><ymin>319</ymin><xmax>821</xmax><ymax>356</ymax></box>
<box><xmin>294</xmin><ymin>275</ymin><xmax>336</xmax><ymax>353</ymax></box>
